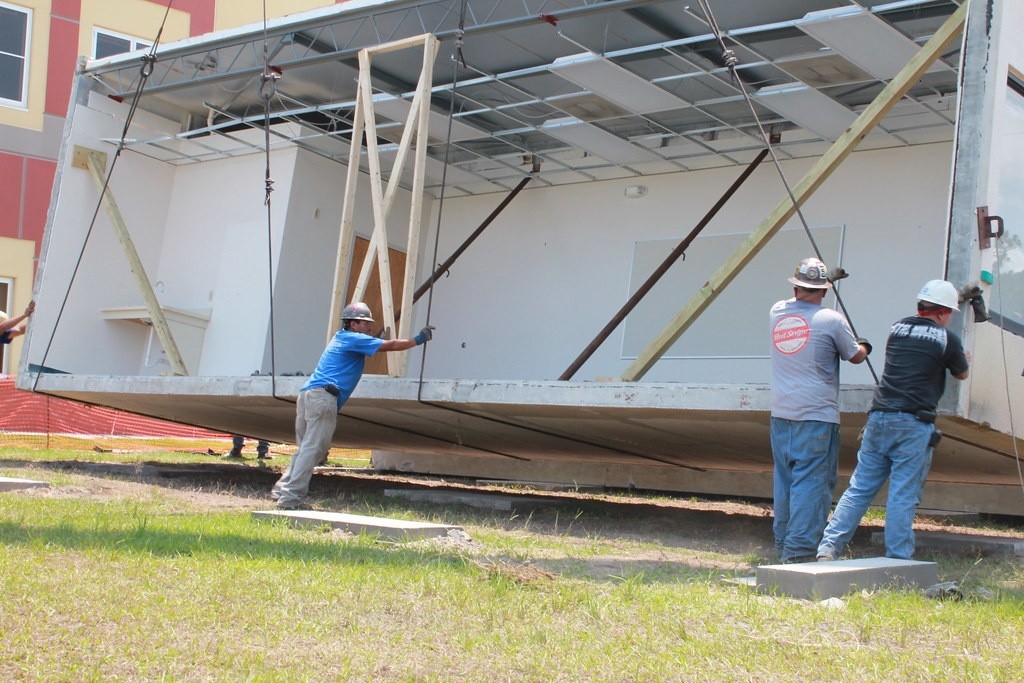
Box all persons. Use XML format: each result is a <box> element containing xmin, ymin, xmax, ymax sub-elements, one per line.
<box><xmin>224</xmin><ymin>435</ymin><xmax>273</xmax><ymax>460</ymax></box>
<box><xmin>270</xmin><ymin>301</ymin><xmax>436</xmax><ymax>512</ymax></box>
<box><xmin>0</xmin><ymin>300</ymin><xmax>36</xmax><ymax>344</ymax></box>
<box><xmin>816</xmin><ymin>279</ymin><xmax>983</xmax><ymax>561</ymax></box>
<box><xmin>770</xmin><ymin>258</ymin><xmax>873</xmax><ymax>565</ymax></box>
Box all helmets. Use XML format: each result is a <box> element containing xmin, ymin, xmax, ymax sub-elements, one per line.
<box><xmin>787</xmin><ymin>257</ymin><xmax>832</xmax><ymax>289</ymax></box>
<box><xmin>341</xmin><ymin>302</ymin><xmax>375</xmax><ymax>322</ymax></box>
<box><xmin>917</xmin><ymin>279</ymin><xmax>960</xmax><ymax>312</ymax></box>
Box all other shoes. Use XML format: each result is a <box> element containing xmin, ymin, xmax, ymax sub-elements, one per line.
<box><xmin>277</xmin><ymin>504</ymin><xmax>314</xmax><ymax>511</ymax></box>
<box><xmin>224</xmin><ymin>449</ymin><xmax>241</xmax><ymax>458</ymax></box>
<box><xmin>257</xmin><ymin>455</ymin><xmax>272</xmax><ymax>459</ymax></box>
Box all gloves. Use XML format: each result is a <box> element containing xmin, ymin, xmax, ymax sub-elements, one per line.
<box><xmin>957</xmin><ymin>281</ymin><xmax>992</xmax><ymax>323</ymax></box>
<box><xmin>376</xmin><ymin>326</ymin><xmax>391</xmax><ymax>340</ymax></box>
<box><xmin>856</xmin><ymin>337</ymin><xmax>872</xmax><ymax>355</ymax></box>
<box><xmin>414</xmin><ymin>326</ymin><xmax>436</xmax><ymax>345</ymax></box>
<box><xmin>830</xmin><ymin>266</ymin><xmax>849</xmax><ymax>281</ymax></box>
